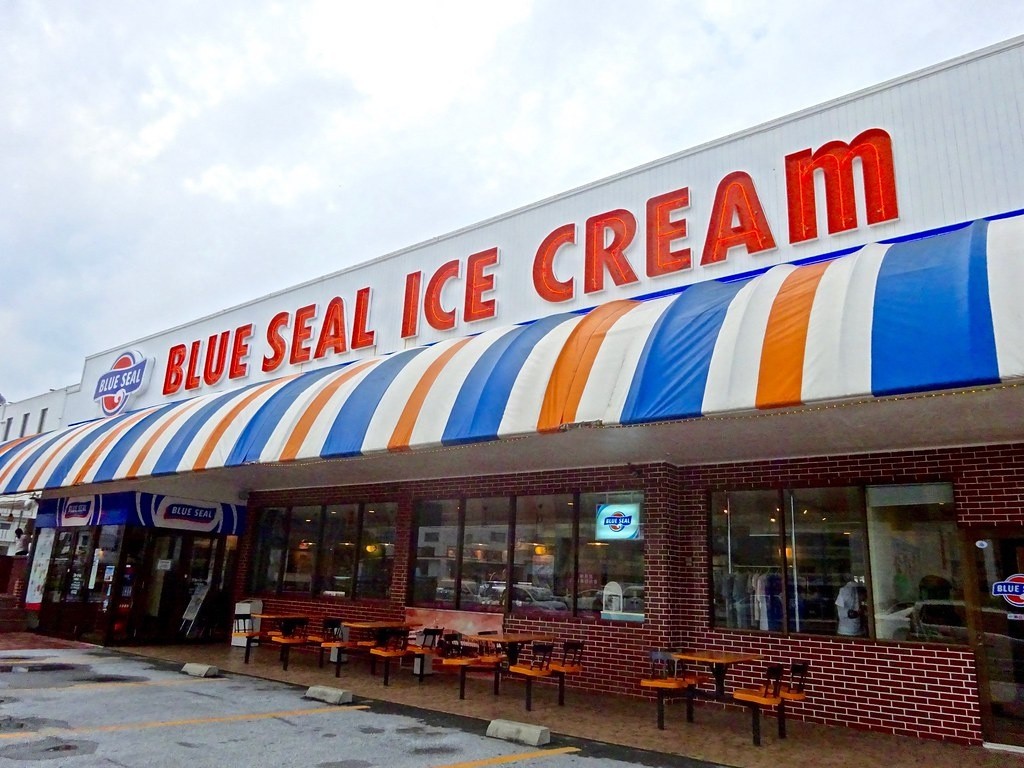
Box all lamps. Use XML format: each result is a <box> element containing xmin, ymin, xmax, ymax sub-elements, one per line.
<box><xmin>24</xmin><ymin>497</ymin><xmax>41</xmax><ymax>511</ymax></box>
<box><xmin>8</xmin><ymin>501</ymin><xmax>16</xmax><ymax>522</ymax></box>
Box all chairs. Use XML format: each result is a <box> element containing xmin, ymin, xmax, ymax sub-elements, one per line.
<box><xmin>443</xmin><ymin>633</ymin><xmax>482</xmax><ymax>700</ymax></box>
<box><xmin>272</xmin><ymin>617</ymin><xmax>308</xmax><ymax>671</ymax></box>
<box><xmin>478</xmin><ymin>630</ymin><xmax>508</xmax><ymax>696</ymax></box>
<box><xmin>322</xmin><ymin>618</ymin><xmax>353</xmax><ymax>678</ymax></box>
<box><xmin>267</xmin><ymin>609</ymin><xmax>293</xmax><ymax>660</ymax></box>
<box><xmin>509</xmin><ymin>643</ymin><xmax>555</xmax><ymax>712</ymax></box>
<box><xmin>731</xmin><ymin>663</ymin><xmax>785</xmax><ymax>747</ymax></box>
<box><xmin>544</xmin><ymin>641</ymin><xmax>587</xmax><ymax>706</ymax></box>
<box><xmin>403</xmin><ymin>627</ymin><xmax>446</xmax><ymax>683</ymax></box>
<box><xmin>358</xmin><ymin>621</ymin><xmax>385</xmax><ymax>678</ymax></box>
<box><xmin>369</xmin><ymin>627</ymin><xmax>411</xmax><ymax>687</ymax></box>
<box><xmin>232</xmin><ymin>613</ymin><xmax>264</xmax><ymax>664</ymax></box>
<box><xmin>309</xmin><ymin>617</ymin><xmax>334</xmax><ymax>669</ymax></box>
<box><xmin>667</xmin><ymin>648</ymin><xmax>708</xmax><ymax>723</ymax></box>
<box><xmin>640</xmin><ymin>650</ymin><xmax>683</xmax><ymax>730</ymax></box>
<box><xmin>759</xmin><ymin>661</ymin><xmax>811</xmax><ymax>740</ymax></box>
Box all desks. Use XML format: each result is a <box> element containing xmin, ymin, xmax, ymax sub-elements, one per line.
<box><xmin>251</xmin><ymin>613</ymin><xmax>326</xmax><ymax>638</ymax></box>
<box><xmin>470</xmin><ymin>634</ymin><xmax>558</xmax><ymax>668</ymax></box>
<box><xmin>671</xmin><ymin>650</ymin><xmax>763</xmax><ymax>708</ymax></box>
<box><xmin>341</xmin><ymin>620</ymin><xmax>423</xmax><ymax>647</ymax></box>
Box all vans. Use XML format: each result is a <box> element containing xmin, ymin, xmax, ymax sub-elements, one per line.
<box><xmin>437</xmin><ymin>580</ymin><xmax>478</xmax><ymax>598</ymax></box>
<box><xmin>512</xmin><ymin>585</ymin><xmax>567</xmax><ymax>613</ymax></box>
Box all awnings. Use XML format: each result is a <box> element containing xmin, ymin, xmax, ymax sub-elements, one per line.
<box><xmin>1</xmin><ymin>207</ymin><xmax>1023</xmax><ymax>499</ymax></box>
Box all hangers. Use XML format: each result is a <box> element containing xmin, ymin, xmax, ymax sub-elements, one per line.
<box><xmin>734</xmin><ymin>564</ymin><xmax>792</xmax><ymax>578</ymax></box>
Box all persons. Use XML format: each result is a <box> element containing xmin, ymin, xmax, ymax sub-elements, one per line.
<box><xmin>15</xmin><ymin>528</ymin><xmax>33</xmax><ymax>555</ymax></box>
<box><xmin>835</xmin><ymin>582</ymin><xmax>862</xmax><ymax>636</ymax></box>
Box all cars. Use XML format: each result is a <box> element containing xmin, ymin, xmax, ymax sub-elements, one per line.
<box><xmin>565</xmin><ymin>589</ymin><xmax>616</xmax><ymax>608</ymax></box>
<box><xmin>480</xmin><ymin>580</ymin><xmax>507</xmax><ymax>597</ymax></box>
